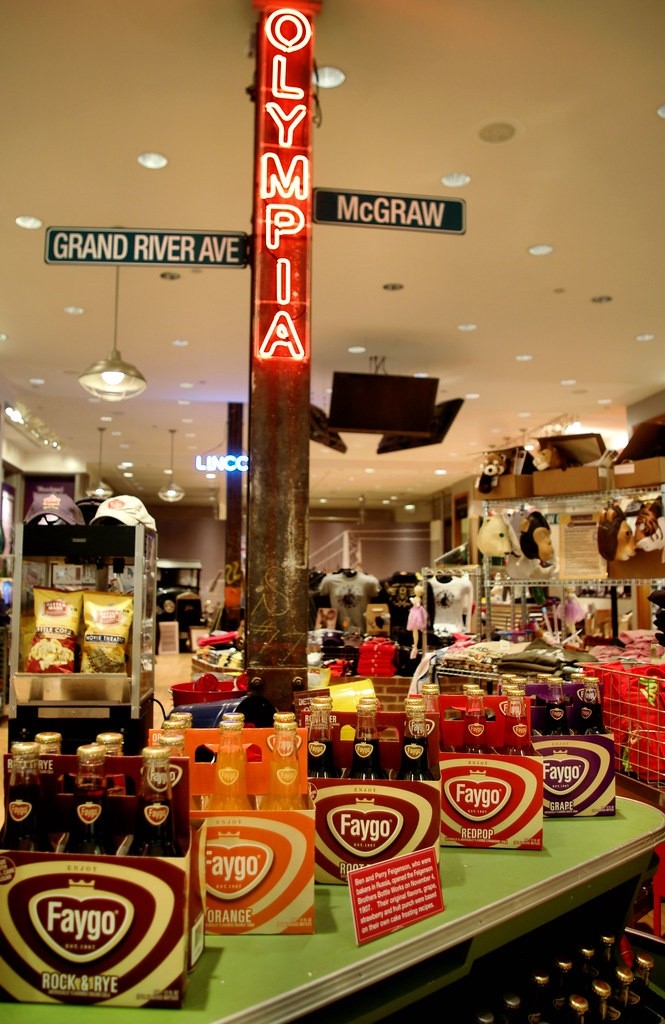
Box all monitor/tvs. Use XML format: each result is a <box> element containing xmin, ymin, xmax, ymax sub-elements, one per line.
<box><xmin>309</xmin><ymin>371</ymin><xmax>464</xmax><ymax>455</ymax></box>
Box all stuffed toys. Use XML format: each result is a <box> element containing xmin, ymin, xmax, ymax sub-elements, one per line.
<box><xmin>634</xmin><ymin>496</ymin><xmax>665</xmax><ymax>551</ymax></box>
<box><xmin>529</xmin><ymin>443</ymin><xmax>582</xmax><ymax>471</ymax></box>
<box><xmin>597</xmin><ymin>498</ymin><xmax>635</xmax><ymax>562</ymax></box>
<box><xmin>479</xmin><ymin>451</ymin><xmax>510</xmax><ymax>494</ymax></box>
<box><xmin>519</xmin><ymin>510</ymin><xmax>555</xmax><ymax>563</ymax></box>
<box><xmin>477</xmin><ymin>516</ymin><xmax>511</xmax><ymax>558</ymax></box>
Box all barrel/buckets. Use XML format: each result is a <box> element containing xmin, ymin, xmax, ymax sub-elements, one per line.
<box><xmin>168</xmin><ymin>680</ymin><xmax>246</xmax><ymax>706</ymax></box>
<box><xmin>325</xmin><ymin>678</ymin><xmax>378</xmax><ymax>711</ymax></box>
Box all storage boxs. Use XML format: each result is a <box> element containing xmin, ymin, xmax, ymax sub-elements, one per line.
<box><xmin>474</xmin><ymin>474</ymin><xmax>534</xmax><ymax>500</ymax></box>
<box><xmin>533</xmin><ymin>466</ymin><xmax>613</xmax><ymax>496</ymax></box>
<box><xmin>613</xmin><ymin>457</ymin><xmax>665</xmax><ymax>489</ymax></box>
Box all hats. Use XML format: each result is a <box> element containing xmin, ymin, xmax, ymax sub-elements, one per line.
<box><xmin>24</xmin><ymin>493</ymin><xmax>85</xmax><ymax>526</ymax></box>
<box><xmin>88</xmin><ymin>494</ymin><xmax>157</xmax><ymax>532</ymax></box>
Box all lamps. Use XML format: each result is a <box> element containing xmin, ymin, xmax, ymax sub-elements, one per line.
<box><xmin>77</xmin><ymin>265</ymin><xmax>147</xmax><ymax>402</ymax></box>
<box><xmin>5</xmin><ymin>402</ymin><xmax>58</xmax><ymax>445</ymax></box>
<box><xmin>85</xmin><ymin>427</ymin><xmax>113</xmax><ymax>498</ymax></box>
<box><xmin>157</xmin><ymin>430</ymin><xmax>185</xmax><ymax>503</ymax></box>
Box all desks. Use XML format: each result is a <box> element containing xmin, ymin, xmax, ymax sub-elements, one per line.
<box><xmin>0</xmin><ymin>795</ymin><xmax>665</xmax><ymax>1024</ymax></box>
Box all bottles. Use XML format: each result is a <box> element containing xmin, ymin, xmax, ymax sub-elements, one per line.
<box><xmin>469</xmin><ymin>932</ymin><xmax>656</xmax><ymax>1024</ymax></box>
<box><xmin>309</xmin><ymin>673</ymin><xmax>608</xmax><ymax>781</ymax></box>
<box><xmin>0</xmin><ymin>711</ymin><xmax>308</xmax><ymax>857</ymax></box>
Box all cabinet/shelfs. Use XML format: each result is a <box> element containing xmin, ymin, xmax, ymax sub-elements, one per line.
<box><xmin>0</xmin><ymin>484</ymin><xmax>665</xmax><ymax>1024</ymax></box>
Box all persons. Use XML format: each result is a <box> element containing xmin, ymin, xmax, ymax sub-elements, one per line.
<box><xmin>319</xmin><ymin>609</ymin><xmax>327</xmax><ymax>628</ymax></box>
<box><xmin>406</xmin><ymin>585</ymin><xmax>429</xmax><ymax>660</ymax></box>
